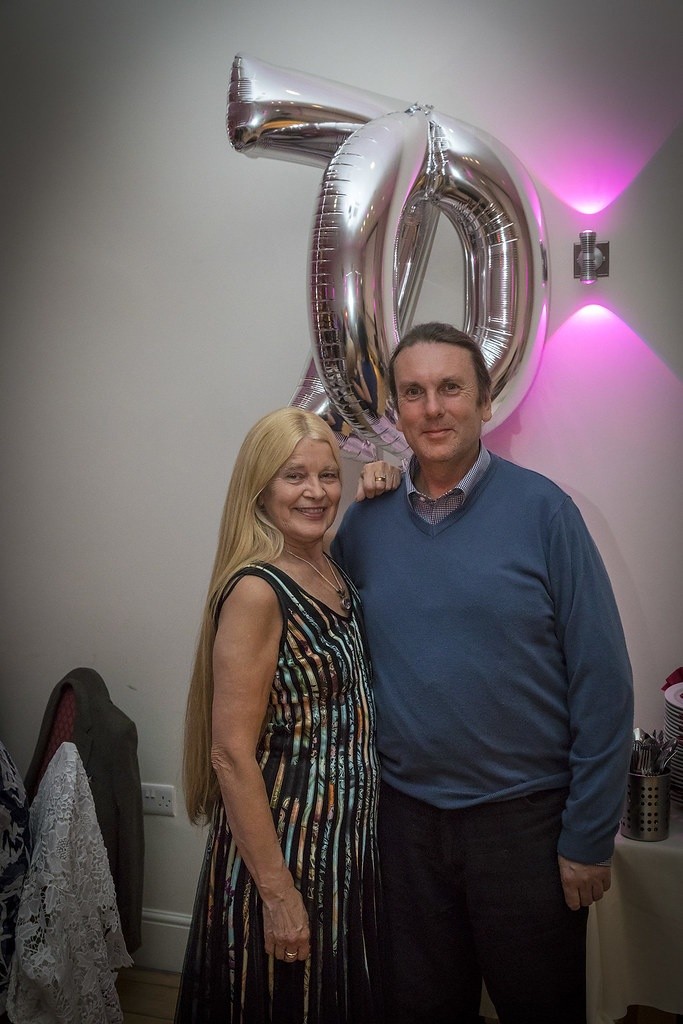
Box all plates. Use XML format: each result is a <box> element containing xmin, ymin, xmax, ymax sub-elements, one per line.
<box><xmin>664</xmin><ymin>682</ymin><xmax>683</xmax><ymax>804</ymax></box>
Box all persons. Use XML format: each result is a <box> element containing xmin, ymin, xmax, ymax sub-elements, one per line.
<box><xmin>169</xmin><ymin>406</ymin><xmax>381</xmax><ymax>1024</ymax></box>
<box><xmin>331</xmin><ymin>323</ymin><xmax>634</xmax><ymax>1024</ymax></box>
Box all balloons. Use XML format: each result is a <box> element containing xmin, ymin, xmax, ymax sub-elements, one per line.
<box><xmin>226</xmin><ymin>49</ymin><xmax>553</xmax><ymax>469</ymax></box>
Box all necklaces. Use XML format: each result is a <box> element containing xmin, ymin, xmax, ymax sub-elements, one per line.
<box><xmin>284</xmin><ymin>548</ymin><xmax>351</xmax><ymax>612</ymax></box>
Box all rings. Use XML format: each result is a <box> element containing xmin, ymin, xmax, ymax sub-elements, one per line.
<box><xmin>375</xmin><ymin>476</ymin><xmax>386</xmax><ymax>481</ymax></box>
<box><xmin>284</xmin><ymin>950</ymin><xmax>297</xmax><ymax>959</ymax></box>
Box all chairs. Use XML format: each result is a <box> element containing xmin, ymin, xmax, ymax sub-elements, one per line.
<box><xmin>22</xmin><ymin>667</ymin><xmax>143</xmax><ymax>853</ymax></box>
<box><xmin>9</xmin><ymin>741</ymin><xmax>134</xmax><ymax>1024</ymax></box>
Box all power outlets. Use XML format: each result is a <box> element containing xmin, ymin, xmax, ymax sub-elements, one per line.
<box><xmin>140</xmin><ymin>783</ymin><xmax>176</xmax><ymax>816</ymax></box>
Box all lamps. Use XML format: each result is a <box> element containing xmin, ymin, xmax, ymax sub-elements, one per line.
<box><xmin>574</xmin><ymin>229</ymin><xmax>610</xmax><ymax>283</ymax></box>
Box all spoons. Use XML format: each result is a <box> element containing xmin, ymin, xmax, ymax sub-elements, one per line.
<box><xmin>627</xmin><ymin>728</ymin><xmax>679</xmax><ymax>775</ymax></box>
